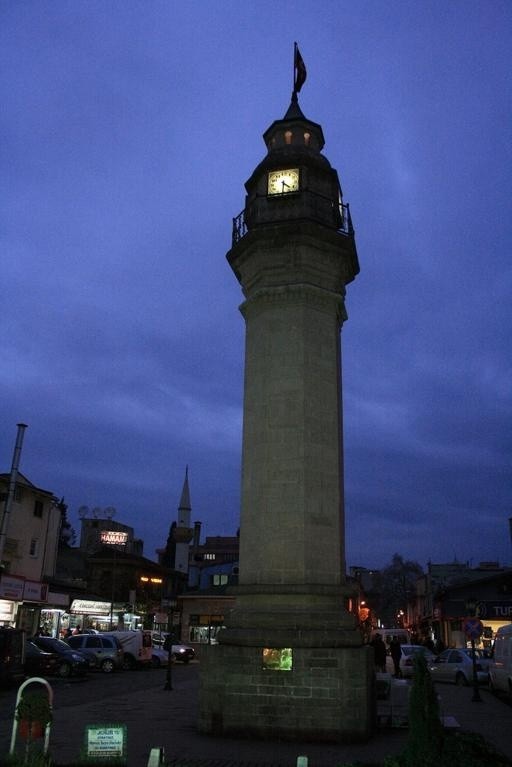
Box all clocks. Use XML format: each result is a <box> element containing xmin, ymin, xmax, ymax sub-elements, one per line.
<box><xmin>267</xmin><ymin>167</ymin><xmax>300</xmax><ymax>196</ymax></box>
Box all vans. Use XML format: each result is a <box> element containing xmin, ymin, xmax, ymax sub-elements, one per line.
<box><xmin>99</xmin><ymin>631</ymin><xmax>152</xmax><ymax>671</ymax></box>
<box><xmin>488</xmin><ymin>623</ymin><xmax>512</xmax><ymax>699</ymax></box>
<box><xmin>369</xmin><ymin>629</ymin><xmax>411</xmax><ymax>654</ymax></box>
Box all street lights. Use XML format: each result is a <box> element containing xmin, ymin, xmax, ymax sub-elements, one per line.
<box><xmin>141</xmin><ymin>577</ymin><xmax>162</xmax><ymax>649</ymax></box>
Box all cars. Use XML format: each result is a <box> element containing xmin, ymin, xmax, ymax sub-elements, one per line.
<box><xmin>133</xmin><ymin>629</ymin><xmax>195</xmax><ymax>669</ymax></box>
<box><xmin>426</xmin><ymin>647</ymin><xmax>490</xmax><ymax>687</ymax></box>
<box><xmin>0</xmin><ymin>637</ymin><xmax>79</xmax><ymax>678</ymax></box>
<box><xmin>385</xmin><ymin>644</ymin><xmax>437</xmax><ymax>681</ymax></box>
<box><xmin>65</xmin><ymin>634</ymin><xmax>124</xmax><ymax>673</ymax></box>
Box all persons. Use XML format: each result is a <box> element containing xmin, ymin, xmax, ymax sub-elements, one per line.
<box><xmin>189</xmin><ymin>626</ymin><xmax>195</xmax><ymax>641</ymax></box>
<box><xmin>410</xmin><ymin>633</ymin><xmax>443</xmax><ymax>653</ymax></box>
<box><xmin>389</xmin><ymin>634</ymin><xmax>403</xmax><ymax>678</ymax></box>
<box><xmin>369</xmin><ymin>632</ymin><xmax>387</xmax><ymax>671</ymax></box>
<box><xmin>40</xmin><ymin>622</ymin><xmax>50</xmax><ymax>635</ymax></box>
<box><xmin>72</xmin><ymin>624</ymin><xmax>82</xmax><ymax>635</ymax></box>
<box><xmin>63</xmin><ymin>627</ymin><xmax>73</xmax><ymax>639</ymax></box>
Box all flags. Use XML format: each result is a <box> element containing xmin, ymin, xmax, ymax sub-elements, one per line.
<box><xmin>293</xmin><ymin>43</ymin><xmax>308</xmax><ymax>95</ymax></box>
